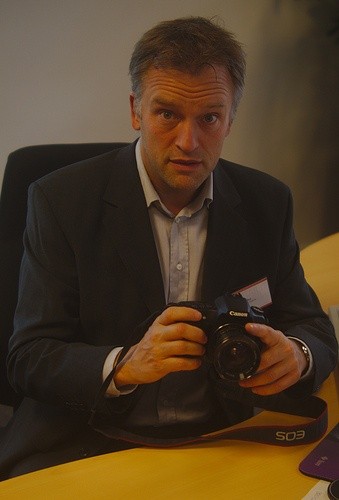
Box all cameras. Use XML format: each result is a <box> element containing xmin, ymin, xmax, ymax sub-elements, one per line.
<box><xmin>176</xmin><ymin>296</ymin><xmax>269</xmax><ymax>384</ymax></box>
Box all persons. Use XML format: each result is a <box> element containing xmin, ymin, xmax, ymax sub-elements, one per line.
<box><xmin>0</xmin><ymin>14</ymin><xmax>339</xmax><ymax>481</ymax></box>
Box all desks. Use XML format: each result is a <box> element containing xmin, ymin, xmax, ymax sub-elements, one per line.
<box><xmin>0</xmin><ymin>230</ymin><xmax>339</xmax><ymax>499</ymax></box>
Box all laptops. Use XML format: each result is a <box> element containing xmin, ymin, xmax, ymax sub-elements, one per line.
<box><xmin>298</xmin><ymin>422</ymin><xmax>339</xmax><ymax>482</ymax></box>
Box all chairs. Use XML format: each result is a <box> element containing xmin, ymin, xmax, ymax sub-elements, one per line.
<box><xmin>0</xmin><ymin>140</ymin><xmax>137</xmax><ymax>428</ymax></box>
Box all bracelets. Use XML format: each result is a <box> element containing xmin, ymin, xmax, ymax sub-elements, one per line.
<box><xmin>299</xmin><ymin>342</ymin><xmax>310</xmax><ymax>363</ymax></box>
